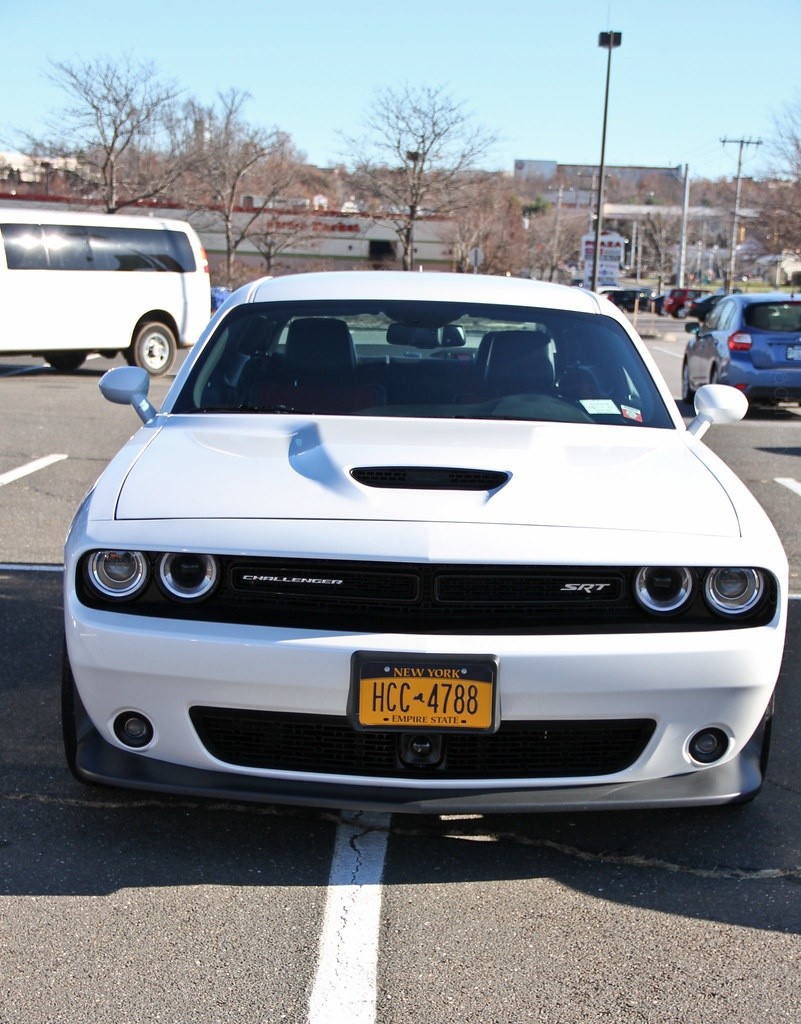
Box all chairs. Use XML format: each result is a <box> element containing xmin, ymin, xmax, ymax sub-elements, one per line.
<box><xmin>455</xmin><ymin>332</ymin><xmax>567</xmax><ymax>405</ymax></box>
<box><xmin>256</xmin><ymin>319</ymin><xmax>380</xmax><ymax>410</ymax></box>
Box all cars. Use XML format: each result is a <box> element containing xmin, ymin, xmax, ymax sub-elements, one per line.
<box><xmin>59</xmin><ymin>271</ymin><xmax>791</xmax><ymax>816</ymax></box>
<box><xmin>682</xmin><ymin>282</ymin><xmax>801</xmax><ymax>413</ymax></box>
<box><xmin>691</xmin><ymin>294</ymin><xmax>726</xmax><ymax>321</ymax></box>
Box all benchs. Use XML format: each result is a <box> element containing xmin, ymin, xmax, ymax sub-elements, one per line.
<box><xmin>264</xmin><ymin>352</ymin><xmax>496</xmax><ymax>408</ymax></box>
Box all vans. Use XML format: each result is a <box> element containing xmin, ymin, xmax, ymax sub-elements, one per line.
<box><xmin>661</xmin><ymin>290</ymin><xmax>710</xmax><ymax>319</ymax></box>
<box><xmin>0</xmin><ymin>208</ymin><xmax>211</xmax><ymax>379</ymax></box>
<box><xmin>608</xmin><ymin>288</ymin><xmax>650</xmax><ymax>312</ymax></box>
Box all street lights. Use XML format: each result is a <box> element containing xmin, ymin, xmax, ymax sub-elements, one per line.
<box><xmin>591</xmin><ymin>32</ymin><xmax>622</xmax><ymax>292</ymax></box>
